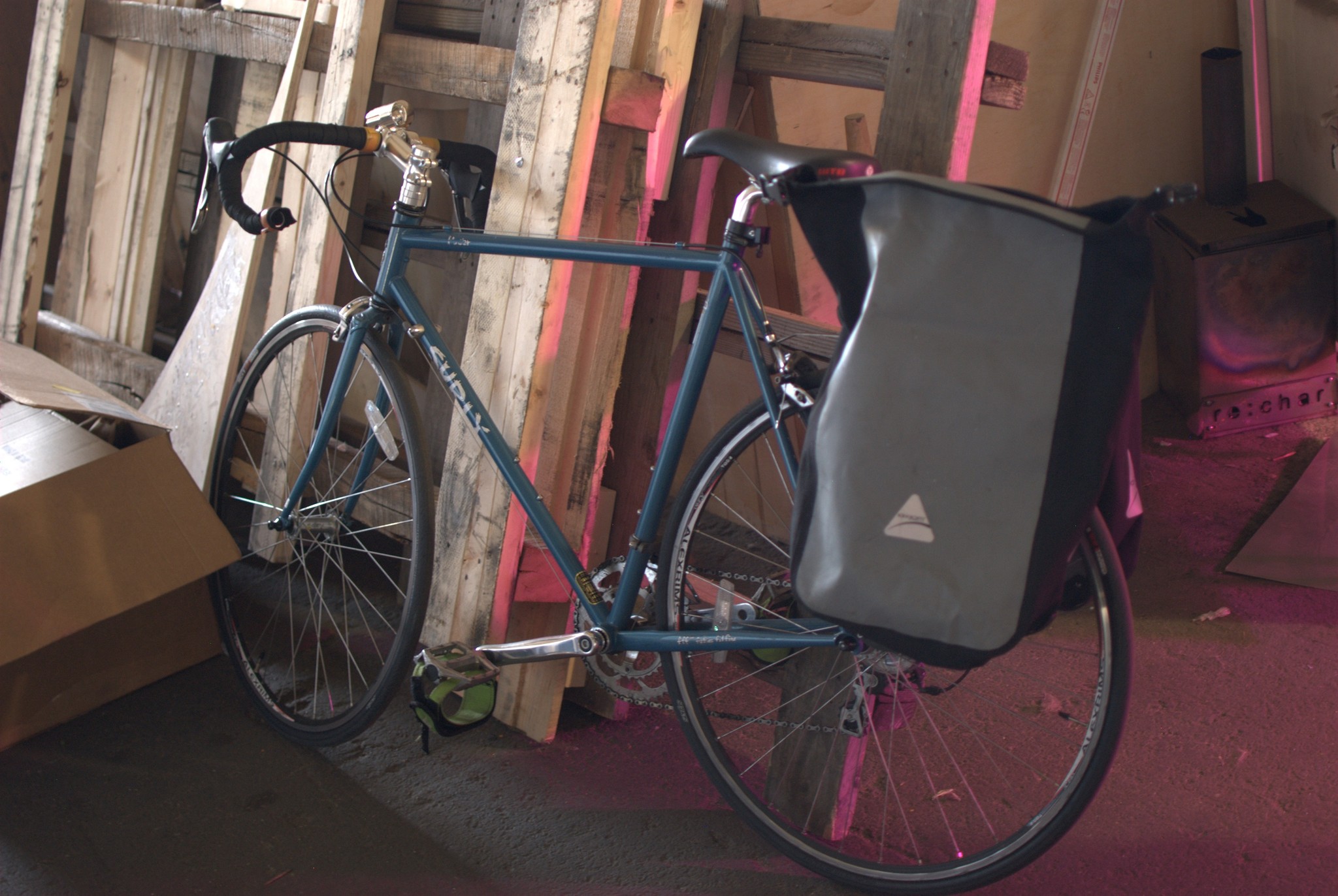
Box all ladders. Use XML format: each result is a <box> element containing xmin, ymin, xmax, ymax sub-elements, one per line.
<box><xmin>564</xmin><ymin>2</ymin><xmax>997</xmax><ymax>837</ymax></box>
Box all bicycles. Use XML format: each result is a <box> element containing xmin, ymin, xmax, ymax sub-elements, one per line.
<box><xmin>199</xmin><ymin>95</ymin><xmax>1140</xmax><ymax>895</ymax></box>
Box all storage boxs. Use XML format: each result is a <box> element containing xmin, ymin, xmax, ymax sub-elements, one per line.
<box><xmin>1151</xmin><ymin>175</ymin><xmax>1337</xmax><ymax>436</ymax></box>
<box><xmin>0</xmin><ymin>339</ymin><xmax>241</xmax><ymax>746</ymax></box>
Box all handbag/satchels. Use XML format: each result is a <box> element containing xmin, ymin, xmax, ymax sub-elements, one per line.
<box><xmin>777</xmin><ymin>164</ymin><xmax>1135</xmax><ymax>669</ymax></box>
